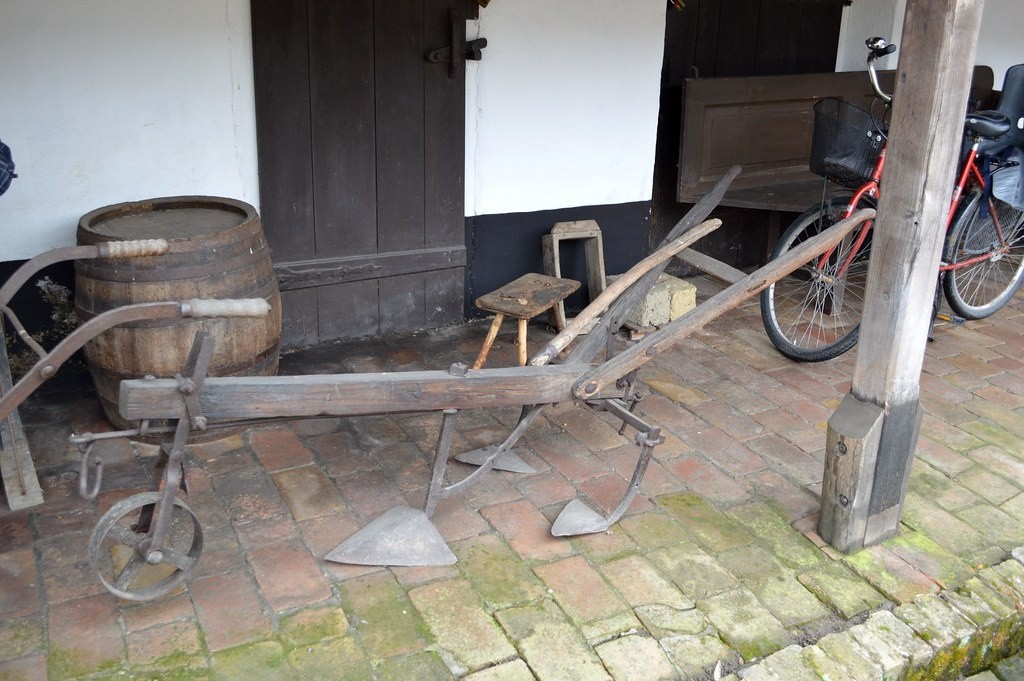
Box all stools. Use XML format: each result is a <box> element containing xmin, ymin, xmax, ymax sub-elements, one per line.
<box><xmin>474</xmin><ymin>272</ymin><xmax>585</xmax><ymax>369</ymax></box>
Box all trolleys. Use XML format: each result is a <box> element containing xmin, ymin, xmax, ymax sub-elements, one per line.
<box><xmin>0</xmin><ymin>237</ymin><xmax>275</xmax><ymax>514</ymax></box>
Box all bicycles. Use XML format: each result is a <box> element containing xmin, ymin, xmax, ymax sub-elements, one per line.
<box><xmin>758</xmin><ymin>37</ymin><xmax>1024</xmax><ymax>361</ymax></box>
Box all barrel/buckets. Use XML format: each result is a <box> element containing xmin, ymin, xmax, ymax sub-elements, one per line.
<box><xmin>76</xmin><ymin>196</ymin><xmax>281</xmax><ymax>445</ymax></box>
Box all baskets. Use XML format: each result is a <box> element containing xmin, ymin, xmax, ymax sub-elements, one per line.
<box><xmin>810</xmin><ymin>98</ymin><xmax>890</xmax><ymax>189</ymax></box>
